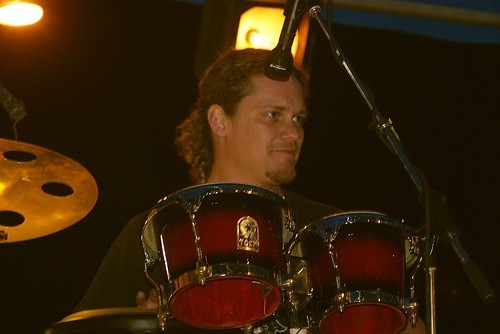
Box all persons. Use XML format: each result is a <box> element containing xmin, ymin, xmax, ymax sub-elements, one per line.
<box><xmin>72</xmin><ymin>48</ymin><xmax>427</xmax><ymax>334</ymax></box>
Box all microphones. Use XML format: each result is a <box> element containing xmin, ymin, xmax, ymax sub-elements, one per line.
<box><xmin>264</xmin><ymin>0</ymin><xmax>306</xmax><ymax>81</ymax></box>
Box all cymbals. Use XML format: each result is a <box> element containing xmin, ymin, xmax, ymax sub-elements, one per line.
<box><xmin>0</xmin><ymin>139</ymin><xmax>99</xmax><ymax>243</ymax></box>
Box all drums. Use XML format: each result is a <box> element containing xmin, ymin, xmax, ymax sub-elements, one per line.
<box><xmin>282</xmin><ymin>210</ymin><xmax>424</xmax><ymax>334</ymax></box>
<box><xmin>43</xmin><ymin>308</ymin><xmax>179</xmax><ymax>334</ymax></box>
<box><xmin>140</xmin><ymin>182</ymin><xmax>288</xmax><ymax>329</ymax></box>
